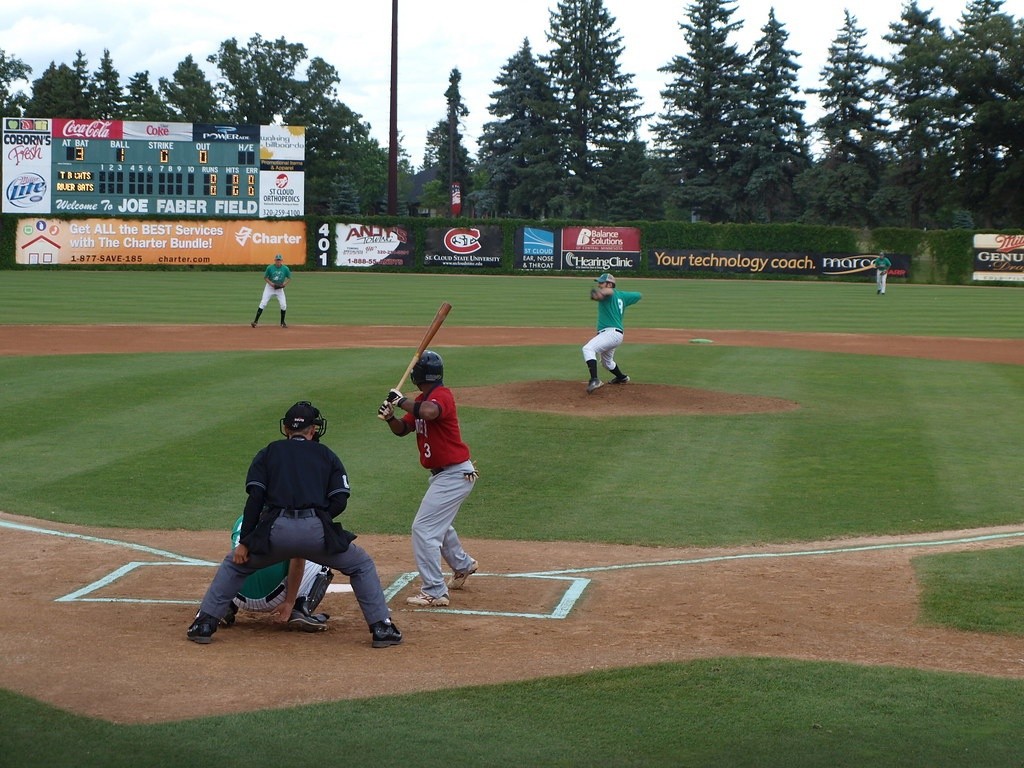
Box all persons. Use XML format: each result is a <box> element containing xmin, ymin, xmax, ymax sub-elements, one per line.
<box><xmin>376</xmin><ymin>351</ymin><xmax>479</xmax><ymax>606</ymax></box>
<box><xmin>582</xmin><ymin>273</ymin><xmax>642</xmax><ymax>395</ymax></box>
<box><xmin>195</xmin><ymin>514</ymin><xmax>334</xmax><ymax>632</ymax></box>
<box><xmin>875</xmin><ymin>252</ymin><xmax>891</xmax><ymax>295</ymax></box>
<box><xmin>186</xmin><ymin>401</ymin><xmax>403</xmax><ymax>648</ymax></box>
<box><xmin>250</xmin><ymin>253</ymin><xmax>292</xmax><ymax>328</ymax></box>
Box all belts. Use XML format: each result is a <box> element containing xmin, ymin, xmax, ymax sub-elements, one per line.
<box><xmin>431</xmin><ymin>467</ymin><xmax>444</xmax><ymax>476</ymax></box>
<box><xmin>237</xmin><ymin>584</ymin><xmax>285</xmax><ymax>603</ymax></box>
<box><xmin>597</xmin><ymin>329</ymin><xmax>623</xmax><ymax>334</ymax></box>
<box><xmin>283</xmin><ymin>509</ymin><xmax>314</xmax><ymax>519</ymax></box>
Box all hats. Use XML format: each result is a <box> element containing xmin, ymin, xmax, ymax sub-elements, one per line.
<box><xmin>274</xmin><ymin>254</ymin><xmax>283</xmax><ymax>260</ymax></box>
<box><xmin>284</xmin><ymin>404</ymin><xmax>322</xmax><ymax>431</ymax></box>
<box><xmin>594</xmin><ymin>273</ymin><xmax>615</xmax><ymax>284</ymax></box>
<box><xmin>879</xmin><ymin>252</ymin><xmax>884</xmax><ymax>256</ymax></box>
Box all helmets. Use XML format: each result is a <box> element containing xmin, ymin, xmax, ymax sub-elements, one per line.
<box><xmin>411</xmin><ymin>350</ymin><xmax>443</xmax><ymax>385</ymax></box>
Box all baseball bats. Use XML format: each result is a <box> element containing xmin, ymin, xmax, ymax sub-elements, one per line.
<box><xmin>378</xmin><ymin>302</ymin><xmax>453</xmax><ymax>421</ymax></box>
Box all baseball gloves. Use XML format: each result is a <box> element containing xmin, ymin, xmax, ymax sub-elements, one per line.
<box><xmin>591</xmin><ymin>289</ymin><xmax>605</xmax><ymax>300</ymax></box>
<box><xmin>274</xmin><ymin>283</ymin><xmax>285</xmax><ymax>289</ymax></box>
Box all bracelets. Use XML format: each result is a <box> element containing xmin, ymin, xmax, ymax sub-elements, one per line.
<box><xmin>413</xmin><ymin>401</ymin><xmax>422</xmax><ymax>419</ymax></box>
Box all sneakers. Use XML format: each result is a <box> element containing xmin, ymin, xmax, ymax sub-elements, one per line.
<box><xmin>406</xmin><ymin>592</ymin><xmax>450</xmax><ymax>606</ymax></box>
<box><xmin>586</xmin><ymin>377</ymin><xmax>605</xmax><ymax>395</ymax></box>
<box><xmin>608</xmin><ymin>375</ymin><xmax>630</xmax><ymax>384</ymax></box>
<box><xmin>186</xmin><ymin>609</ymin><xmax>219</xmax><ymax>644</ymax></box>
<box><xmin>217</xmin><ymin>605</ymin><xmax>235</xmax><ymax>628</ymax></box>
<box><xmin>286</xmin><ymin>609</ymin><xmax>331</xmax><ymax>633</ymax></box>
<box><xmin>370</xmin><ymin>617</ymin><xmax>402</xmax><ymax>648</ymax></box>
<box><xmin>446</xmin><ymin>556</ymin><xmax>480</xmax><ymax>590</ymax></box>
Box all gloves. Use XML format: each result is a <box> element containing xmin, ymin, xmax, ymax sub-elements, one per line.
<box><xmin>387</xmin><ymin>388</ymin><xmax>408</xmax><ymax>408</ymax></box>
<box><xmin>272</xmin><ymin>282</ymin><xmax>285</xmax><ymax>289</ymax></box>
<box><xmin>377</xmin><ymin>400</ymin><xmax>395</xmax><ymax>423</ymax></box>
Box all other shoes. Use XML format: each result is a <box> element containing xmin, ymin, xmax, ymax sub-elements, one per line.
<box><xmin>251</xmin><ymin>322</ymin><xmax>257</xmax><ymax>328</ymax></box>
<box><xmin>877</xmin><ymin>290</ymin><xmax>884</xmax><ymax>295</ymax></box>
<box><xmin>280</xmin><ymin>322</ymin><xmax>286</xmax><ymax>327</ymax></box>
